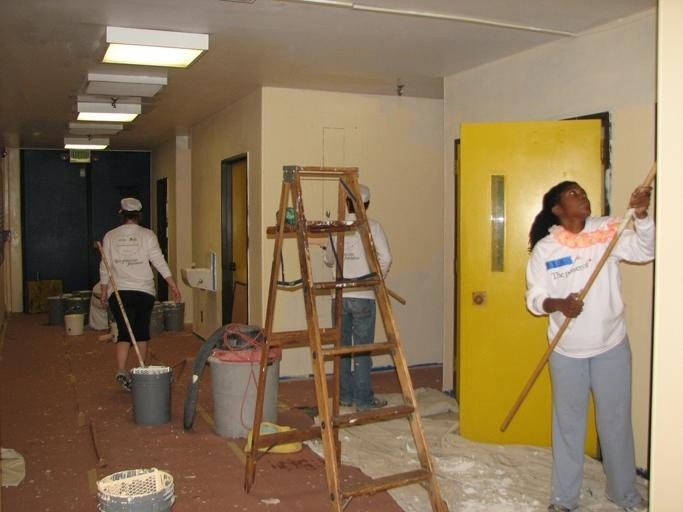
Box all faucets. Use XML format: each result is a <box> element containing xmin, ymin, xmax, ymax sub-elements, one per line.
<box><xmin>191</xmin><ymin>264</ymin><xmax>197</xmax><ymax>269</ymax></box>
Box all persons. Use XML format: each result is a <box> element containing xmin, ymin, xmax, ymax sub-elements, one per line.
<box><xmin>524</xmin><ymin>181</ymin><xmax>656</xmax><ymax>512</ymax></box>
<box><xmin>100</xmin><ymin>198</ymin><xmax>181</xmax><ymax>392</ymax></box>
<box><xmin>322</xmin><ymin>185</ymin><xmax>392</xmax><ymax>411</ymax></box>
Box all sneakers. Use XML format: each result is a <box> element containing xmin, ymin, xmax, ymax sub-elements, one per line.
<box><xmin>355</xmin><ymin>398</ymin><xmax>388</xmax><ymax>411</ymax></box>
<box><xmin>604</xmin><ymin>492</ymin><xmax>649</xmax><ymax>512</ymax></box>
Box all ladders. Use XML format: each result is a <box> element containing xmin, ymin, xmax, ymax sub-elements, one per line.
<box><xmin>245</xmin><ymin>166</ymin><xmax>450</xmax><ymax>512</ymax></box>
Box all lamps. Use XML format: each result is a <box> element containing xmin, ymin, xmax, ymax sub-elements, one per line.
<box><xmin>60</xmin><ymin>133</ymin><xmax>111</xmax><ymax>150</ymax></box>
<box><xmin>92</xmin><ymin>24</ymin><xmax>211</xmax><ymax>69</ymax></box>
<box><xmin>80</xmin><ymin>69</ymin><xmax>171</xmax><ymax>98</ymax></box>
<box><xmin>66</xmin><ymin>118</ymin><xmax>125</xmax><ymax>137</ymax></box>
<box><xmin>73</xmin><ymin>94</ymin><xmax>142</xmax><ymax>123</ymax></box>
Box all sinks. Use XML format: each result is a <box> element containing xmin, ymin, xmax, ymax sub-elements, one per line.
<box><xmin>181</xmin><ymin>268</ymin><xmax>213</xmax><ymax>291</ymax></box>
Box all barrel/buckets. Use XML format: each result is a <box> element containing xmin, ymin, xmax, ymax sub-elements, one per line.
<box><xmin>46</xmin><ymin>289</ymin><xmax>185</xmax><ymax>336</ymax></box>
<box><xmin>94</xmin><ymin>467</ymin><xmax>177</xmax><ymax>512</ymax></box>
<box><xmin>129</xmin><ymin>366</ymin><xmax>173</xmax><ymax>426</ymax></box>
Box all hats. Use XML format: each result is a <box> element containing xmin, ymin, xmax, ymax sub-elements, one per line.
<box><xmin>348</xmin><ymin>184</ymin><xmax>370</xmax><ymax>202</ymax></box>
<box><xmin>115</xmin><ymin>370</ymin><xmax>133</xmax><ymax>392</ymax></box>
<box><xmin>120</xmin><ymin>198</ymin><xmax>142</xmax><ymax>213</ymax></box>
